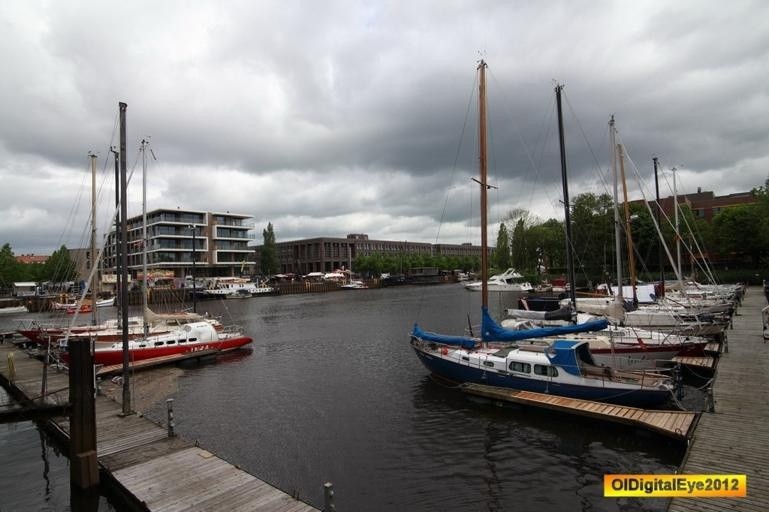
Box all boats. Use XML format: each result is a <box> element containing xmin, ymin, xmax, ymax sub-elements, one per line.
<box><xmin>341</xmin><ymin>280</ymin><xmax>369</xmax><ymax>290</ymax></box>
<box><xmin>191</xmin><ymin>280</ymin><xmax>273</xmax><ymax>300</ymax></box>
